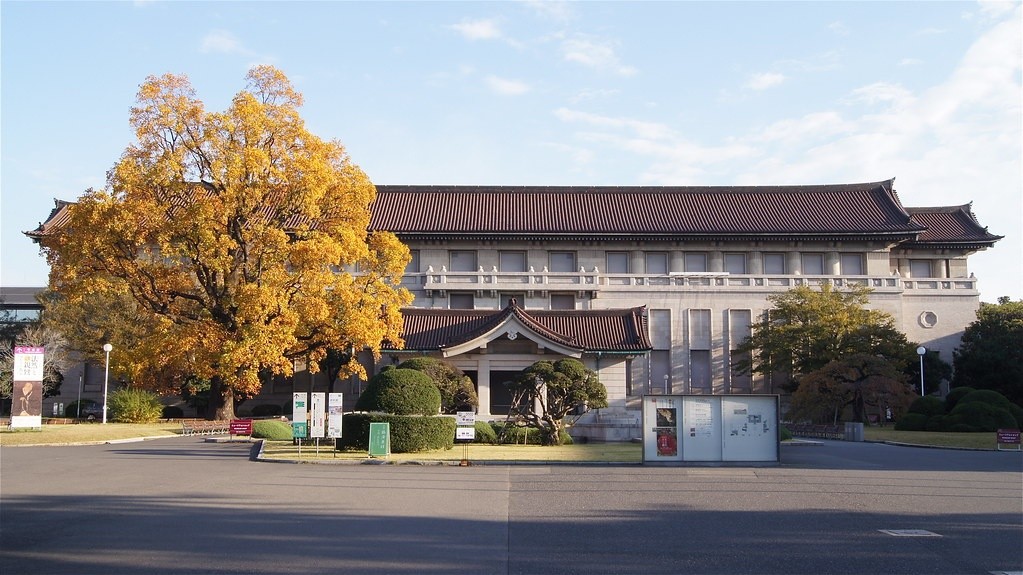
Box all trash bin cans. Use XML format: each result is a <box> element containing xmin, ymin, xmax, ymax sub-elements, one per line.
<box><xmin>845</xmin><ymin>422</ymin><xmax>864</xmax><ymax>442</ymax></box>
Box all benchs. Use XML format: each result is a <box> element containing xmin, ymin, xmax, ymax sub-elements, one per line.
<box><xmin>784</xmin><ymin>423</ymin><xmax>839</xmax><ymax>440</ymax></box>
<box><xmin>46</xmin><ymin>418</ymin><xmax>80</xmax><ymax>424</ymax></box>
<box><xmin>183</xmin><ymin>420</ymin><xmax>230</xmax><ymax>437</ymax></box>
